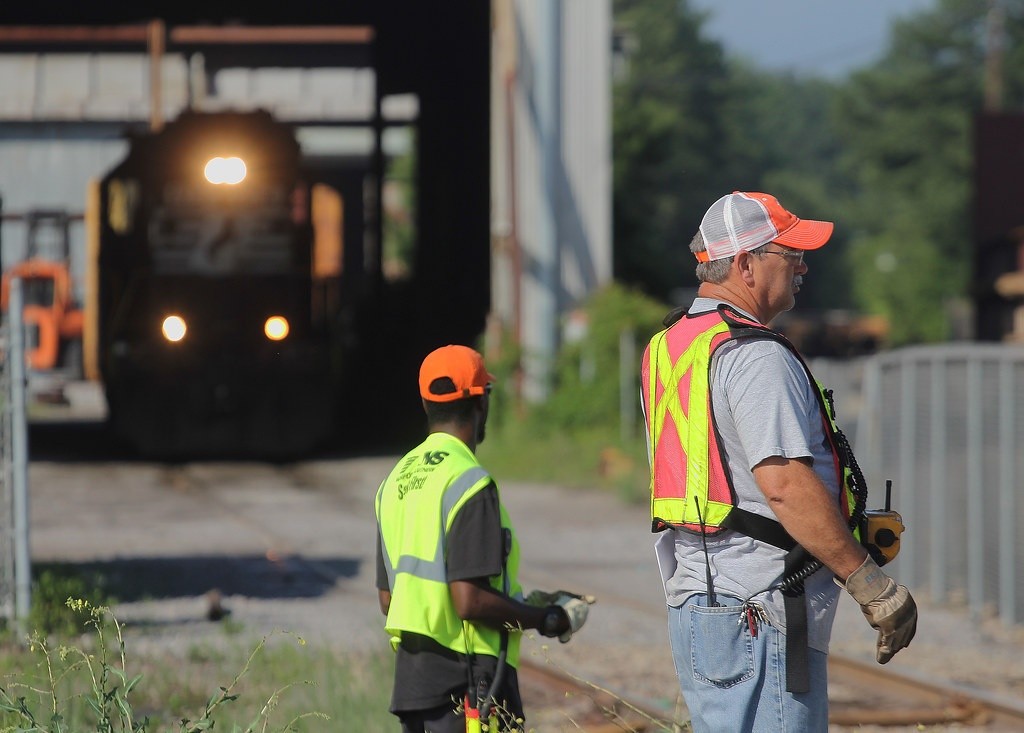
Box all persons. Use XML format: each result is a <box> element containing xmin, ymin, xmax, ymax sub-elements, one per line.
<box><xmin>640</xmin><ymin>190</ymin><xmax>919</xmax><ymax>733</ymax></box>
<box><xmin>375</xmin><ymin>344</ymin><xmax>596</xmax><ymax>733</ymax></box>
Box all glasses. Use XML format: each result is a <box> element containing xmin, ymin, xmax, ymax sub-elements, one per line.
<box><xmin>761</xmin><ymin>248</ymin><xmax>804</xmax><ymax>266</ymax></box>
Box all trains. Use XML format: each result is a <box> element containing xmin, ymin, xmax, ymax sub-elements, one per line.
<box><xmin>80</xmin><ymin>104</ymin><xmax>348</xmax><ymax>461</ymax></box>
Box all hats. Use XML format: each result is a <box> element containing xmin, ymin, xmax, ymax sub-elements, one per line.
<box><xmin>695</xmin><ymin>191</ymin><xmax>833</xmax><ymax>263</ymax></box>
<box><xmin>419</xmin><ymin>344</ymin><xmax>496</xmax><ymax>402</ymax></box>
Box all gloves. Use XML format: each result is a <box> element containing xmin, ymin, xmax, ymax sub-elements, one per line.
<box><xmin>545</xmin><ymin>590</ymin><xmax>596</xmax><ymax>645</ymax></box>
<box><xmin>832</xmin><ymin>554</ymin><xmax>918</xmax><ymax>665</ymax></box>
